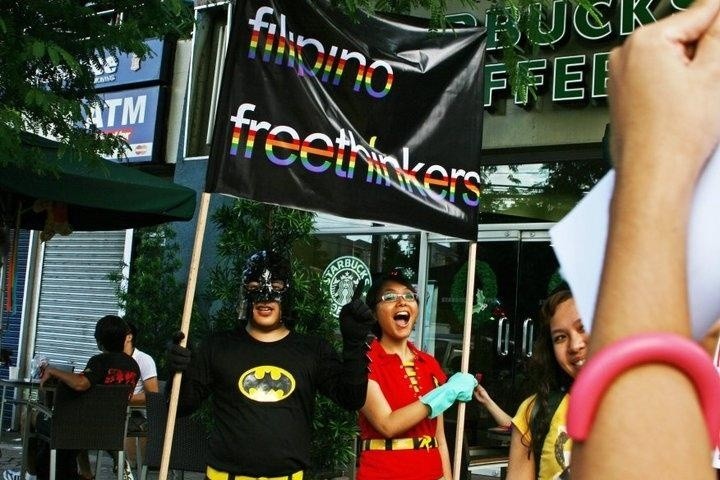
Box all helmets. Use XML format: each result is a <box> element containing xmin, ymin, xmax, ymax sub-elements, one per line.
<box><xmin>236</xmin><ymin>251</ymin><xmax>293</xmax><ymax>323</ymax></box>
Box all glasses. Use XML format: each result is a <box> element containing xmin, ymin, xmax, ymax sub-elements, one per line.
<box><xmin>378</xmin><ymin>291</ymin><xmax>420</xmax><ymax>303</ymax></box>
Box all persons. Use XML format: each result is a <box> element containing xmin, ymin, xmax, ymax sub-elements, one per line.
<box><xmin>162</xmin><ymin>242</ymin><xmax>379</xmax><ymax>480</ymax></box>
<box><xmin>75</xmin><ymin>315</ymin><xmax>159</xmax><ymax>480</ymax></box>
<box><xmin>355</xmin><ymin>267</ymin><xmax>480</xmax><ymax>480</ymax></box>
<box><xmin>562</xmin><ymin>0</ymin><xmax>720</xmax><ymax>480</ymax></box>
<box><xmin>502</xmin><ymin>285</ymin><xmax>593</xmax><ymax>480</ymax></box>
<box><xmin>474</xmin><ymin>381</ymin><xmax>513</xmax><ymax>430</ymax></box>
<box><xmin>3</xmin><ymin>314</ymin><xmax>141</xmax><ymax>480</ymax></box>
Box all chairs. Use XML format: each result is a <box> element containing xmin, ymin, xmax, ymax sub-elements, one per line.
<box><xmin>1</xmin><ymin>363</ymin><xmax>211</xmax><ymax>480</ymax></box>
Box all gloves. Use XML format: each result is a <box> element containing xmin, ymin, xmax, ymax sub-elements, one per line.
<box><xmin>339</xmin><ymin>279</ymin><xmax>377</xmax><ymax>351</ymax></box>
<box><xmin>420</xmin><ymin>372</ymin><xmax>478</xmax><ymax>421</ymax></box>
<box><xmin>163</xmin><ymin>331</ymin><xmax>192</xmax><ymax>381</ymax></box>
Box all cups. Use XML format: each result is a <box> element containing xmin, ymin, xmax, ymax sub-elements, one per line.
<box><xmin>9</xmin><ymin>367</ymin><xmax>19</xmax><ymax>380</ymax></box>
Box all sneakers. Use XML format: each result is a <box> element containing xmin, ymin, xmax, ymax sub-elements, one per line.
<box><xmin>3</xmin><ymin>469</ymin><xmax>26</xmax><ymax>480</ymax></box>
<box><xmin>114</xmin><ymin>460</ymin><xmax>133</xmax><ymax>480</ymax></box>
